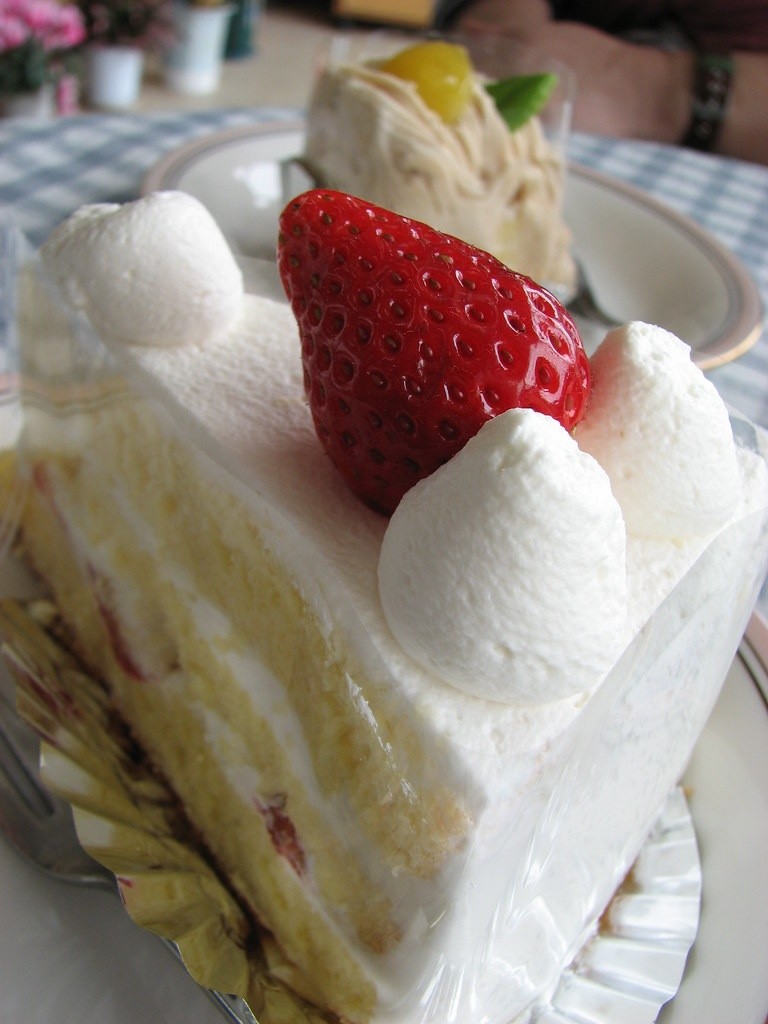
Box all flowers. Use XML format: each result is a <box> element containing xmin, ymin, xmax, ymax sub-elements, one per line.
<box><xmin>0</xmin><ymin>0</ymin><xmax>92</xmax><ymax>93</ymax></box>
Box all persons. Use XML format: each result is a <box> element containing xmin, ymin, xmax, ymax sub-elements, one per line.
<box><xmin>429</xmin><ymin>0</ymin><xmax>768</xmax><ymax>168</ymax></box>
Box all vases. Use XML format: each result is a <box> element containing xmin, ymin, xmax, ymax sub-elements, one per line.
<box><xmin>0</xmin><ymin>5</ymin><xmax>238</xmax><ymax>117</ymax></box>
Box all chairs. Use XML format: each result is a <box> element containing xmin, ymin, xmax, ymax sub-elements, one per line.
<box><xmin>429</xmin><ymin>0</ymin><xmax>735</xmax><ymax>155</ymax></box>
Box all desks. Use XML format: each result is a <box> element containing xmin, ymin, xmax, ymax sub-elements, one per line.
<box><xmin>0</xmin><ymin>102</ymin><xmax>768</xmax><ymax>626</ymax></box>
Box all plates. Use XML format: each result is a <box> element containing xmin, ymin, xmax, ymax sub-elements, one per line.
<box><xmin>0</xmin><ymin>604</ymin><xmax>768</xmax><ymax>1024</ymax></box>
<box><xmin>140</xmin><ymin>116</ymin><xmax>766</xmax><ymax>371</ymax></box>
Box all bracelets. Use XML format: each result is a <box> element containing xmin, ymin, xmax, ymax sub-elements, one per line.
<box><xmin>680</xmin><ymin>46</ymin><xmax>735</xmax><ymax>153</ymax></box>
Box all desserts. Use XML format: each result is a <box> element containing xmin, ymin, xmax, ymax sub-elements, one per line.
<box><xmin>303</xmin><ymin>40</ymin><xmax>576</xmax><ymax>305</ymax></box>
<box><xmin>20</xmin><ymin>187</ymin><xmax>768</xmax><ymax>1024</ymax></box>
